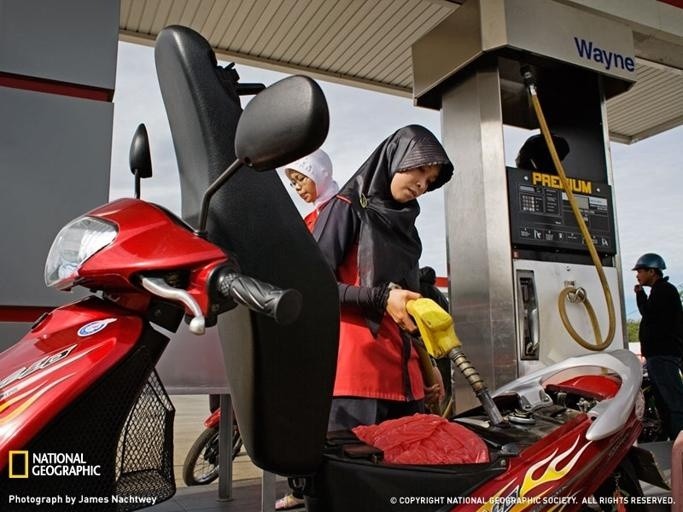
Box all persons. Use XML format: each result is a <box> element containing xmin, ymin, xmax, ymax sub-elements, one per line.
<box><xmin>272</xmin><ymin>148</ymin><xmax>340</xmax><ymax>510</ymax></box>
<box><xmin>631</xmin><ymin>253</ymin><xmax>683</xmax><ymax>440</ymax></box>
<box><xmin>417</xmin><ymin>262</ymin><xmax>451</xmax><ymax>398</ymax></box>
<box><xmin>312</xmin><ymin>123</ymin><xmax>453</xmax><ymax>439</ymax></box>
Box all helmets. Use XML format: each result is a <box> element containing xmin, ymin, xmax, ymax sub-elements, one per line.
<box><xmin>630</xmin><ymin>251</ymin><xmax>667</xmax><ymax>272</ymax></box>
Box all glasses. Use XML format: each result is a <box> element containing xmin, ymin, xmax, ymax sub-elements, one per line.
<box><xmin>288</xmin><ymin>174</ymin><xmax>308</xmax><ymax>188</ymax></box>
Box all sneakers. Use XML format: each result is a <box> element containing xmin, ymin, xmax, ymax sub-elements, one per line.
<box><xmin>274</xmin><ymin>492</ymin><xmax>305</xmax><ymax>510</ymax></box>
